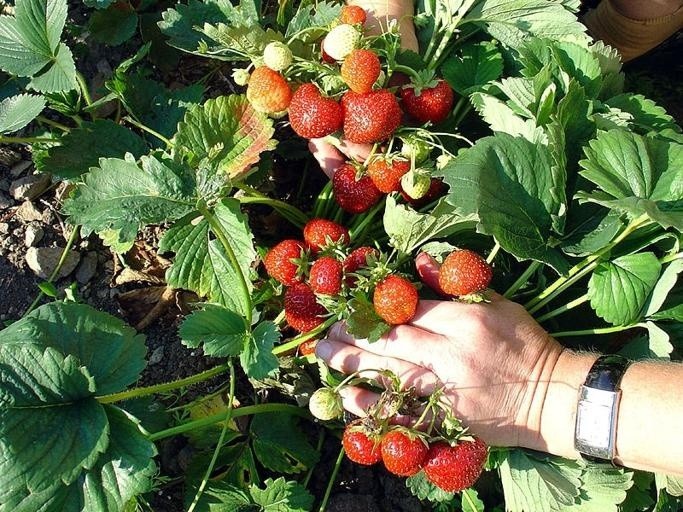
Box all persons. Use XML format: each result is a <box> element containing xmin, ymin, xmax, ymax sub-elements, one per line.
<box><xmin>350</xmin><ymin>1</ymin><xmax>682</xmax><ymax>128</ymax></box>
<box><xmin>313</xmin><ymin>252</ymin><xmax>683</xmax><ymax>479</ymax></box>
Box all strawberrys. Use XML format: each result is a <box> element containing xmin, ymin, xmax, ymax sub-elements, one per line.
<box><xmin>246</xmin><ymin>6</ymin><xmax>492</xmax><ymax>493</ymax></box>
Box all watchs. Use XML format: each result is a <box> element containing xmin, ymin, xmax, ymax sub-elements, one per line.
<box><xmin>571</xmin><ymin>353</ymin><xmax>632</xmax><ymax>469</ymax></box>
